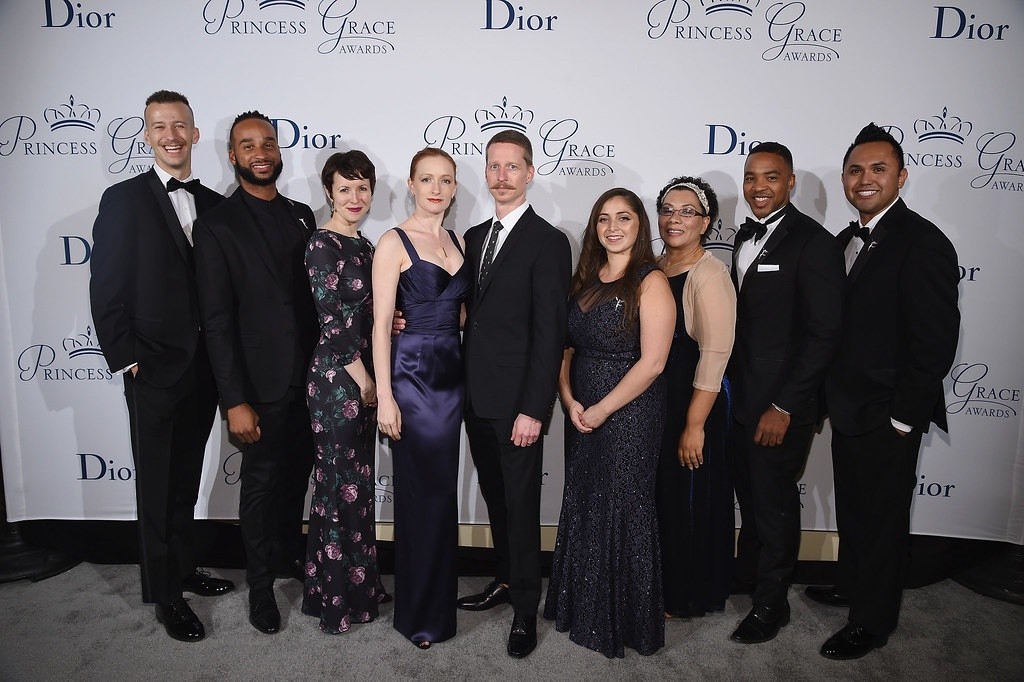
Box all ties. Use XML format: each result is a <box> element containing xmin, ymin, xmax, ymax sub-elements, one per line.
<box><xmin>478</xmin><ymin>221</ymin><xmax>504</xmax><ymax>292</ymax></box>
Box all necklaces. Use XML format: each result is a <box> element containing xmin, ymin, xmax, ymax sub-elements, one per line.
<box><xmin>413</xmin><ymin>212</ymin><xmax>448</xmax><ymax>257</ymax></box>
<box><xmin>663</xmin><ymin>247</ymin><xmax>703</xmax><ymax>273</ymax></box>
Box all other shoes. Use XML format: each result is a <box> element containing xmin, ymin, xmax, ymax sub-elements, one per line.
<box><xmin>380</xmin><ymin>594</ymin><xmax>393</xmax><ymax>604</ymax></box>
<box><xmin>414</xmin><ymin>639</ymin><xmax>431</xmax><ymax>650</ymax></box>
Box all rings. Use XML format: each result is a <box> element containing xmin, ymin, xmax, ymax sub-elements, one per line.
<box><xmin>527</xmin><ymin>443</ymin><xmax>531</xmax><ymax>445</ymax></box>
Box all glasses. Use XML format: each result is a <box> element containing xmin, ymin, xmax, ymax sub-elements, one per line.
<box><xmin>658</xmin><ymin>205</ymin><xmax>708</xmax><ymax>217</ymax></box>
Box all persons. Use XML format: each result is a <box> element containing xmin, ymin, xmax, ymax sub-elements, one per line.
<box><xmin>731</xmin><ymin>143</ymin><xmax>846</xmax><ymax>647</ymax></box>
<box><xmin>651</xmin><ymin>176</ymin><xmax>738</xmax><ymax>618</ymax></box>
<box><xmin>806</xmin><ymin>123</ymin><xmax>960</xmax><ymax>660</ymax></box>
<box><xmin>89</xmin><ymin>91</ymin><xmax>229</xmax><ymax>641</ymax></box>
<box><xmin>304</xmin><ymin>150</ymin><xmax>376</xmax><ymax>631</ymax></box>
<box><xmin>542</xmin><ymin>188</ymin><xmax>666</xmax><ymax>659</ymax></box>
<box><xmin>372</xmin><ymin>149</ymin><xmax>475</xmax><ymax>649</ymax></box>
<box><xmin>192</xmin><ymin>110</ymin><xmax>317</xmax><ymax>633</ymax></box>
<box><xmin>393</xmin><ymin>130</ymin><xmax>572</xmax><ymax>657</ymax></box>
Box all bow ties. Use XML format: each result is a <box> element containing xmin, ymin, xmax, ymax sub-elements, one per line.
<box><xmin>739</xmin><ymin>203</ymin><xmax>792</xmax><ymax>246</ymax></box>
<box><xmin>850</xmin><ymin>220</ymin><xmax>870</xmax><ymax>244</ymax></box>
<box><xmin>166</xmin><ymin>177</ymin><xmax>200</xmax><ymax>197</ymax></box>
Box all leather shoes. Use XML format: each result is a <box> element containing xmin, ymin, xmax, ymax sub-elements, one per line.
<box><xmin>731</xmin><ymin>600</ymin><xmax>791</xmax><ymax>643</ymax></box>
<box><xmin>821</xmin><ymin>623</ymin><xmax>888</xmax><ymax>661</ymax></box>
<box><xmin>277</xmin><ymin>542</ymin><xmax>309</xmax><ymax>581</ymax></box>
<box><xmin>457</xmin><ymin>581</ymin><xmax>514</xmax><ymax>611</ymax></box>
<box><xmin>155</xmin><ymin>595</ymin><xmax>205</xmax><ymax>642</ymax></box>
<box><xmin>248</xmin><ymin>582</ymin><xmax>280</xmax><ymax>636</ymax></box>
<box><xmin>174</xmin><ymin>562</ymin><xmax>236</xmax><ymax>596</ymax></box>
<box><xmin>805</xmin><ymin>584</ymin><xmax>851</xmax><ymax>607</ymax></box>
<box><xmin>506</xmin><ymin>609</ymin><xmax>538</xmax><ymax>658</ymax></box>
